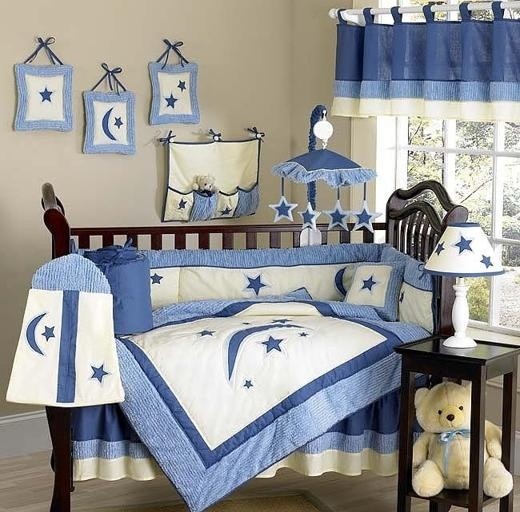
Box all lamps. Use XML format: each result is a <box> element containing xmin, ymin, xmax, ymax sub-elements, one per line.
<box><xmin>424</xmin><ymin>222</ymin><xmax>503</xmax><ymax>349</ymax></box>
<box><xmin>268</xmin><ymin>104</ymin><xmax>382</xmax><ymax>232</ymax></box>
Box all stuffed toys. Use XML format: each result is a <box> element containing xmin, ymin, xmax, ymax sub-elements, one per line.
<box><xmin>193</xmin><ymin>173</ymin><xmax>217</xmax><ymax>194</ymax></box>
<box><xmin>411</xmin><ymin>382</ymin><xmax>515</xmax><ymax>500</ymax></box>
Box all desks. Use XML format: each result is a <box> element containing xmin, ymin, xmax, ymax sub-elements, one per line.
<box><xmin>394</xmin><ymin>335</ymin><xmax>518</xmax><ymax>510</ymax></box>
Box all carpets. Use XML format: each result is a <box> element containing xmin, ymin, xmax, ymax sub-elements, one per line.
<box><xmin>114</xmin><ymin>487</ymin><xmax>337</xmax><ymax>510</ymax></box>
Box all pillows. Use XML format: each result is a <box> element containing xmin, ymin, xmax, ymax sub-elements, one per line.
<box><xmin>342</xmin><ymin>262</ymin><xmax>405</xmax><ymax>322</ymax></box>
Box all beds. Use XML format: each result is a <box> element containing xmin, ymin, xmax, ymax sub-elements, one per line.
<box><xmin>37</xmin><ymin>180</ymin><xmax>468</xmax><ymax>512</ymax></box>
